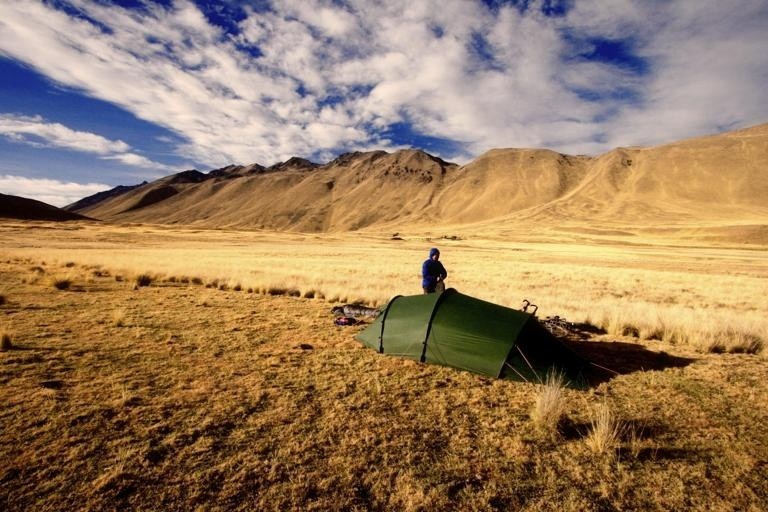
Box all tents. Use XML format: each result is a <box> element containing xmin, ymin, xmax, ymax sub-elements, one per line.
<box><xmin>352</xmin><ymin>288</ymin><xmax>563</xmax><ymax>380</ymax></box>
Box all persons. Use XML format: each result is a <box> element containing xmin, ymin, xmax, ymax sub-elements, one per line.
<box><xmin>422</xmin><ymin>248</ymin><xmax>447</xmax><ymax>294</ymax></box>
